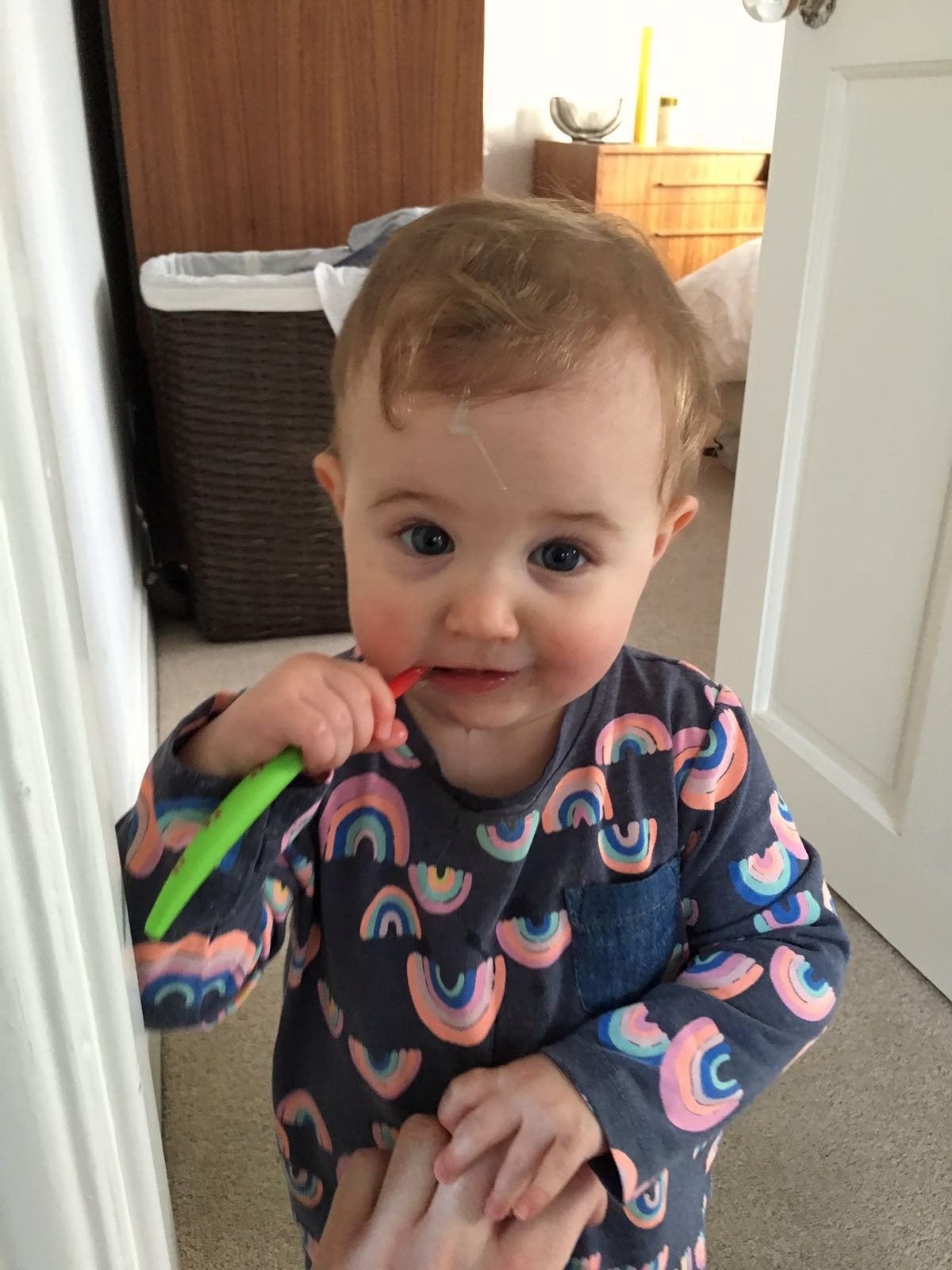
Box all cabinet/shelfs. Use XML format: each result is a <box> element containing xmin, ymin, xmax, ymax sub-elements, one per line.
<box><xmin>535</xmin><ymin>140</ymin><xmax>767</xmax><ymax>280</ymax></box>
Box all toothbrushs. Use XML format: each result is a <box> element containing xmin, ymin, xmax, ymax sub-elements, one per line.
<box><xmin>144</xmin><ymin>667</ymin><xmax>433</xmax><ymax>943</ymax></box>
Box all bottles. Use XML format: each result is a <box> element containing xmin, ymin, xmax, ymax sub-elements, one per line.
<box><xmin>657</xmin><ymin>97</ymin><xmax>678</xmax><ymax>144</ymax></box>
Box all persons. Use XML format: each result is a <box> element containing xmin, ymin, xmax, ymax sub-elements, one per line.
<box><xmin>110</xmin><ymin>193</ymin><xmax>847</xmax><ymax>1270</ymax></box>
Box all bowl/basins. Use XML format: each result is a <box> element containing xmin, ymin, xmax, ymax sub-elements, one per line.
<box><xmin>550</xmin><ymin>96</ymin><xmax>624</xmax><ymax>141</ymax></box>
